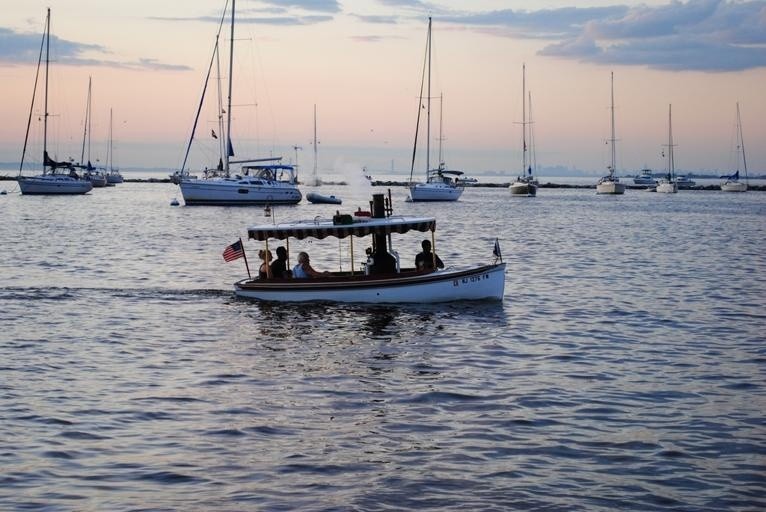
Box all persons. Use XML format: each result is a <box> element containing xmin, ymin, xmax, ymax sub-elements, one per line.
<box><xmin>415</xmin><ymin>240</ymin><xmax>444</xmax><ymax>273</ymax></box>
<box><xmin>258</xmin><ymin>246</ymin><xmax>329</xmax><ymax>280</ymax></box>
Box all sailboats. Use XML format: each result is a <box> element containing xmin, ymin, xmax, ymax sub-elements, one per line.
<box><xmin>408</xmin><ymin>18</ymin><xmax>477</xmax><ymax>201</ymax></box>
<box><xmin>18</xmin><ymin>10</ymin><xmax>123</xmax><ymax>196</ymax></box>
<box><xmin>509</xmin><ymin>65</ymin><xmax>540</xmax><ymax>197</ymax></box>
<box><xmin>172</xmin><ymin>1</ymin><xmax>320</xmax><ymax>203</ymax></box>
<box><xmin>656</xmin><ymin>104</ymin><xmax>677</xmax><ymax>194</ymax></box>
<box><xmin>718</xmin><ymin>101</ymin><xmax>750</xmax><ymax>192</ymax></box>
<box><xmin>598</xmin><ymin>71</ymin><xmax>625</xmax><ymax>195</ymax></box>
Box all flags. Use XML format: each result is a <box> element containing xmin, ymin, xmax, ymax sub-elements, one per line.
<box><xmin>223</xmin><ymin>241</ymin><xmax>244</xmax><ymax>262</ymax></box>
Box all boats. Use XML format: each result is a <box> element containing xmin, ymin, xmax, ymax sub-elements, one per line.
<box><xmin>673</xmin><ymin>172</ymin><xmax>695</xmax><ymax>187</ymax></box>
<box><xmin>234</xmin><ymin>189</ymin><xmax>506</xmax><ymax>305</ymax></box>
<box><xmin>306</xmin><ymin>191</ymin><xmax>341</xmax><ymax>204</ymax></box>
<box><xmin>634</xmin><ymin>168</ymin><xmax>656</xmax><ymax>184</ymax></box>
